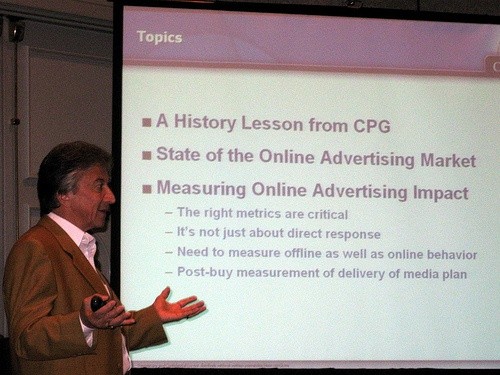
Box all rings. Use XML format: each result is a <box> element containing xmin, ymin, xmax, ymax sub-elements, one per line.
<box><xmin>105</xmin><ymin>321</ymin><xmax>113</xmax><ymax>329</ymax></box>
<box><xmin>187</xmin><ymin>316</ymin><xmax>188</xmax><ymax>319</ymax></box>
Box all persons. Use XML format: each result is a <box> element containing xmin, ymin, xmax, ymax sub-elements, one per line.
<box><xmin>2</xmin><ymin>141</ymin><xmax>208</xmax><ymax>375</ymax></box>
